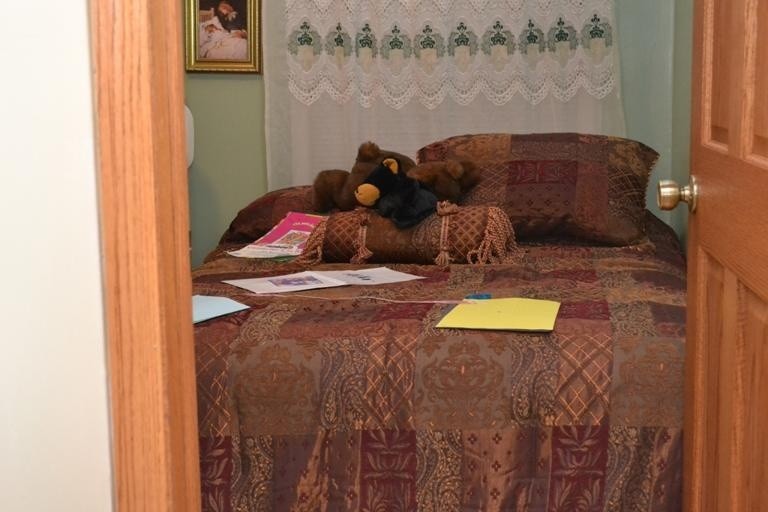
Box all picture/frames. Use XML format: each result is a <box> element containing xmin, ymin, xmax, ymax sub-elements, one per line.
<box><xmin>182</xmin><ymin>0</ymin><xmax>262</xmax><ymax>76</ymax></box>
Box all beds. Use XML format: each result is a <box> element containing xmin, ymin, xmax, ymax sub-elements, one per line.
<box><xmin>192</xmin><ymin>207</ymin><xmax>688</xmax><ymax>511</ymax></box>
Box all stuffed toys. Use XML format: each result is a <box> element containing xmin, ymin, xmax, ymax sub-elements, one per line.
<box><xmin>311</xmin><ymin>141</ymin><xmax>480</xmax><ymax>215</ymax></box>
<box><xmin>354</xmin><ymin>158</ymin><xmax>440</xmax><ymax>228</ymax></box>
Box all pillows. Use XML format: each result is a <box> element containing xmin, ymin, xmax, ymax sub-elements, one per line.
<box><xmin>294</xmin><ymin>207</ymin><xmax>529</xmax><ymax>265</ymax></box>
<box><xmin>221</xmin><ymin>184</ymin><xmax>324</xmax><ymax>243</ymax></box>
<box><xmin>417</xmin><ymin>132</ymin><xmax>659</xmax><ymax>247</ymax></box>
